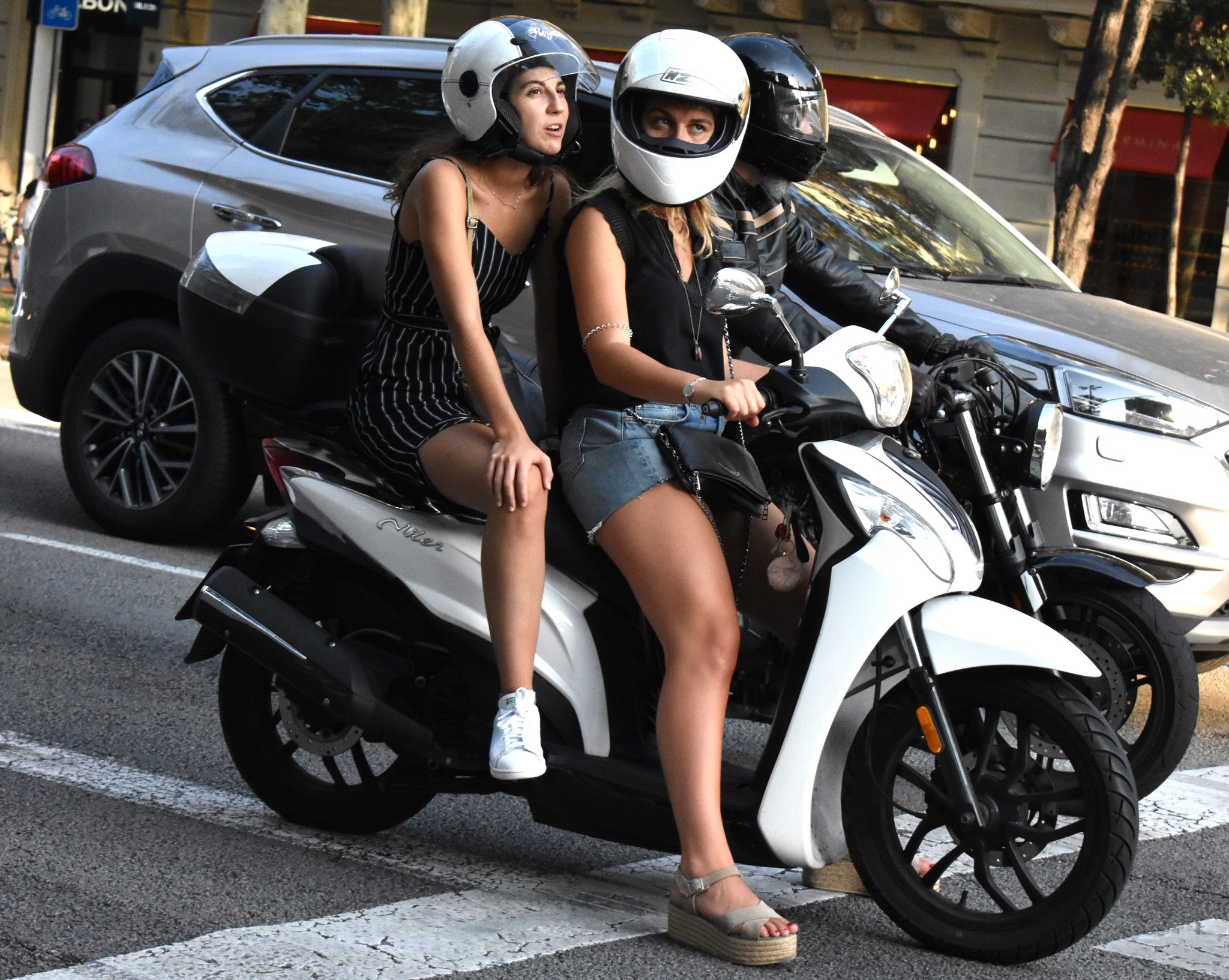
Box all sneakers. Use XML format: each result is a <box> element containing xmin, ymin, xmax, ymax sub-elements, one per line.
<box><xmin>488</xmin><ymin>686</ymin><xmax>548</xmax><ymax>781</ymax></box>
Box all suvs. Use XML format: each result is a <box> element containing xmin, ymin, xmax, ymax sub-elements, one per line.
<box><xmin>7</xmin><ymin>36</ymin><xmax>1228</xmax><ymax>679</ymax></box>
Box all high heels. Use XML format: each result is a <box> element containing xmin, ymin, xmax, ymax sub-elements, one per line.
<box><xmin>666</xmin><ymin>864</ymin><xmax>798</xmax><ymax>966</ymax></box>
<box><xmin>802</xmin><ymin>853</ymin><xmax>940</xmax><ymax>896</ymax></box>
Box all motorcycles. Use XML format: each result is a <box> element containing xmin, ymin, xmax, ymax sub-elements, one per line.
<box><xmin>722</xmin><ymin>331</ymin><xmax>1203</xmax><ymax>818</ymax></box>
<box><xmin>173</xmin><ymin>230</ymin><xmax>1140</xmax><ymax>963</ymax></box>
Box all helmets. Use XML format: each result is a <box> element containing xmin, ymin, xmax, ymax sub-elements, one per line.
<box><xmin>440</xmin><ymin>14</ymin><xmax>601</xmax><ymax>172</ymax></box>
<box><xmin>718</xmin><ymin>31</ymin><xmax>830</xmax><ymax>183</ymax></box>
<box><xmin>608</xmin><ymin>27</ymin><xmax>751</xmax><ymax>207</ymax></box>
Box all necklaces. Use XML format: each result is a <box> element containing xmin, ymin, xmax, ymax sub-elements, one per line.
<box><xmin>649</xmin><ymin>207</ymin><xmax>708</xmax><ymax>362</ymax></box>
<box><xmin>478</xmin><ymin>161</ymin><xmax>530</xmax><ymax>210</ymax></box>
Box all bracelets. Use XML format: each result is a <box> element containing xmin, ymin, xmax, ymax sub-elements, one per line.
<box><xmin>15</xmin><ymin>223</ymin><xmax>22</xmax><ymax>227</ymax></box>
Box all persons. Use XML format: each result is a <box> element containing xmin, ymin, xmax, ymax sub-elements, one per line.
<box><xmin>538</xmin><ymin>26</ymin><xmax>943</xmax><ymax>963</ymax></box>
<box><xmin>10</xmin><ymin>177</ymin><xmax>39</xmax><ymax>240</ymax></box>
<box><xmin>346</xmin><ymin>9</ymin><xmax>595</xmax><ymax>785</ymax></box>
<box><xmin>647</xmin><ymin>31</ymin><xmax>1010</xmax><ymax>532</ymax></box>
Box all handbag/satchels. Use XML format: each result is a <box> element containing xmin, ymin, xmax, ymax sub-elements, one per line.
<box><xmin>652</xmin><ymin>425</ymin><xmax>774</xmax><ymax>521</ymax></box>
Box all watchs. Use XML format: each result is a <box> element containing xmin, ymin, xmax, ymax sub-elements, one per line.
<box><xmin>683</xmin><ymin>375</ymin><xmax>708</xmax><ymax>408</ymax></box>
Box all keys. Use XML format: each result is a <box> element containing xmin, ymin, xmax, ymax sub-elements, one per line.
<box><xmin>762</xmin><ymin>489</ymin><xmax>820</xmax><ymax>592</ymax></box>
